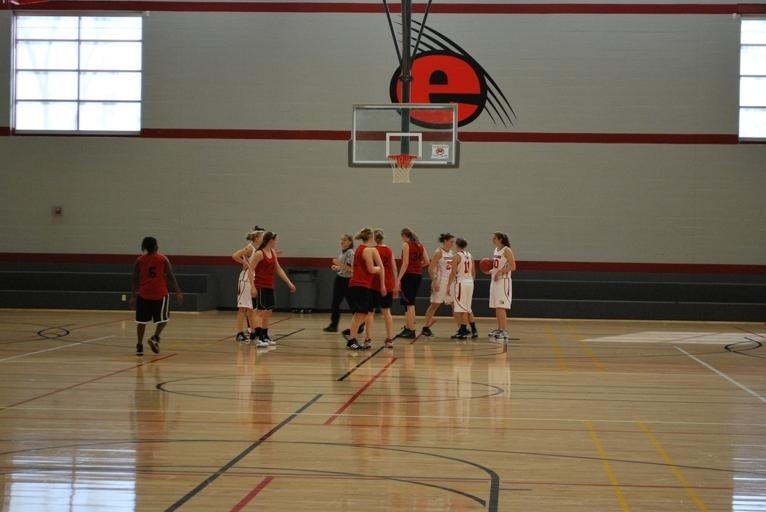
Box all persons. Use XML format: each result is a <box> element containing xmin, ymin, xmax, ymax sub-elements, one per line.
<box><xmin>130</xmin><ymin>238</ymin><xmax>183</xmax><ymax>355</ymax></box>
<box><xmin>323</xmin><ymin>234</ymin><xmax>364</xmax><ymax>333</ymax></box>
<box><xmin>397</xmin><ymin>229</ymin><xmax>430</xmax><ymax>338</ymax></box>
<box><xmin>245</xmin><ymin>226</ymin><xmax>266</xmax><ymax>333</ymax></box>
<box><xmin>447</xmin><ymin>238</ymin><xmax>478</xmax><ymax>339</ymax></box>
<box><xmin>234</xmin><ymin>342</ymin><xmax>273</xmax><ymax>466</ymax></box>
<box><xmin>248</xmin><ymin>232</ymin><xmax>296</xmax><ymax>347</ymax></box>
<box><xmin>129</xmin><ymin>357</ymin><xmax>180</xmax><ymax>480</ymax></box>
<box><xmin>422</xmin><ymin>233</ymin><xmax>470</xmax><ymax>336</ymax></box>
<box><xmin>323</xmin><ymin>332</ymin><xmax>511</xmax><ymax>463</ymax></box>
<box><xmin>341</xmin><ymin>227</ymin><xmax>381</xmax><ymax>350</ymax></box>
<box><xmin>480</xmin><ymin>233</ymin><xmax>516</xmax><ymax>338</ymax></box>
<box><xmin>232</xmin><ymin>230</ymin><xmax>265</xmax><ymax>342</ymax></box>
<box><xmin>364</xmin><ymin>230</ymin><xmax>398</xmax><ymax>348</ymax></box>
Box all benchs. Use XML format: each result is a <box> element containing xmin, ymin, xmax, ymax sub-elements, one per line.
<box><xmin>0</xmin><ymin>260</ymin><xmax>219</xmax><ymax>312</ymax></box>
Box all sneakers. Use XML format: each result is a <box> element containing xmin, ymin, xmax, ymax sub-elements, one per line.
<box><xmin>488</xmin><ymin>330</ymin><xmax>508</xmax><ymax>338</ymax></box>
<box><xmin>398</xmin><ymin>327</ymin><xmax>431</xmax><ymax>338</ymax></box>
<box><xmin>324</xmin><ymin>326</ymin><xmax>372</xmax><ymax>350</ymax></box>
<box><xmin>237</xmin><ymin>330</ymin><xmax>276</xmax><ymax>347</ymax></box>
<box><xmin>136</xmin><ymin>337</ymin><xmax>160</xmax><ymax>354</ymax></box>
<box><xmin>385</xmin><ymin>339</ymin><xmax>392</xmax><ymax>347</ymax></box>
<box><xmin>455</xmin><ymin>328</ymin><xmax>478</xmax><ymax>338</ymax></box>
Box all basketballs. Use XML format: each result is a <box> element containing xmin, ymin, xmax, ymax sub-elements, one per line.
<box><xmin>480</xmin><ymin>257</ymin><xmax>493</xmax><ymax>271</ymax></box>
